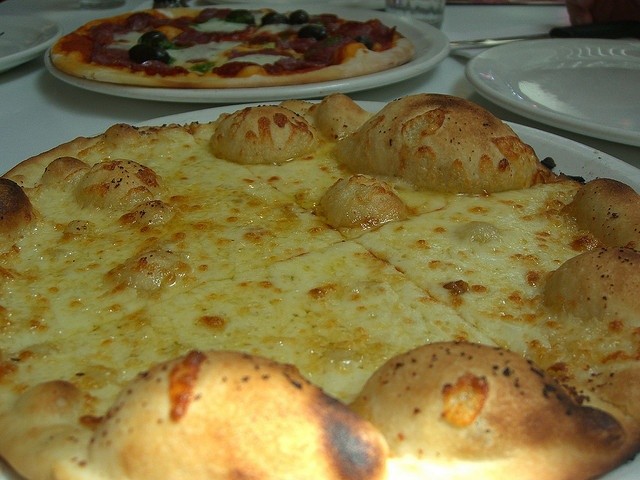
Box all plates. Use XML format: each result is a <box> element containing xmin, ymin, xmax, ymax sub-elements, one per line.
<box><xmin>464</xmin><ymin>36</ymin><xmax>640</xmax><ymax>149</ymax></box>
<box><xmin>0</xmin><ymin>9</ymin><xmax>62</xmax><ymax>74</ymax></box>
<box><xmin>44</xmin><ymin>0</ymin><xmax>452</xmax><ymax>102</ymax></box>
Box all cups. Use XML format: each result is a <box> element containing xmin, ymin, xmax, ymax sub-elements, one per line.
<box><xmin>383</xmin><ymin>0</ymin><xmax>446</xmax><ymax>30</ymax></box>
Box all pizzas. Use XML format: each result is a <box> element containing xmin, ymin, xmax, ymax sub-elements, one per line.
<box><xmin>49</xmin><ymin>7</ymin><xmax>416</xmax><ymax>90</ymax></box>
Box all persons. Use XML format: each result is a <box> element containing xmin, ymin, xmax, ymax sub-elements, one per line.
<box><xmin>563</xmin><ymin>0</ymin><xmax>640</xmax><ymax>26</ymax></box>
